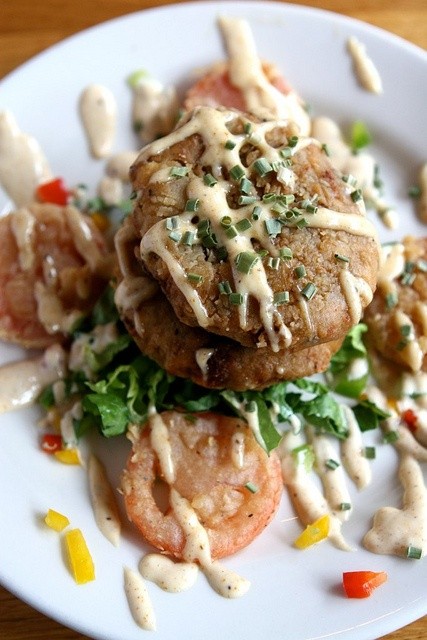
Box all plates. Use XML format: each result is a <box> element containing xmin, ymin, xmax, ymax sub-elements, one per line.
<box><xmin>0</xmin><ymin>1</ymin><xmax>426</xmax><ymax>640</ymax></box>
